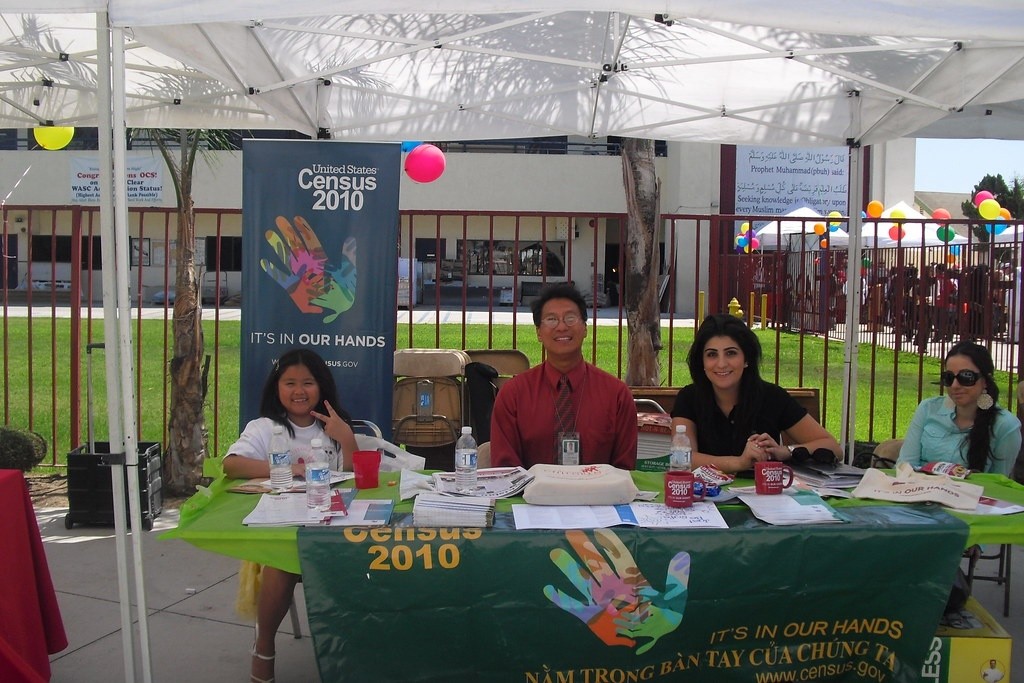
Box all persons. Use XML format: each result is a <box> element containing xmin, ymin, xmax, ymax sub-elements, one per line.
<box><xmin>895</xmin><ymin>341</ymin><xmax>1022</xmax><ymax>559</ymax></box>
<box><xmin>569</xmin><ymin>443</ymin><xmax>573</xmax><ymax>450</ymax></box>
<box><xmin>490</xmin><ymin>280</ymin><xmax>638</xmax><ymax>470</ymax></box>
<box><xmin>223</xmin><ymin>349</ymin><xmax>360</xmax><ymax>683</ymax></box>
<box><xmin>670</xmin><ymin>314</ymin><xmax>844</xmax><ymax>474</ymax></box>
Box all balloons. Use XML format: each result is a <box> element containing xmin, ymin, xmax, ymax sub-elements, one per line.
<box><xmin>975</xmin><ymin>190</ymin><xmax>1011</xmax><ymax>236</ymax></box>
<box><xmin>401</xmin><ymin>142</ymin><xmax>446</xmax><ymax>183</ymax></box>
<box><xmin>814</xmin><ymin>201</ymin><xmax>906</xmax><ymax>248</ymax></box>
<box><xmin>33</xmin><ymin>127</ymin><xmax>75</xmax><ymax>150</ymax></box>
<box><xmin>932</xmin><ymin>208</ymin><xmax>961</xmax><ymax>263</ymax></box>
<box><xmin>734</xmin><ymin>222</ymin><xmax>759</xmax><ymax>253</ymax></box>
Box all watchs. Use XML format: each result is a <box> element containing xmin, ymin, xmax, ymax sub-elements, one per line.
<box><xmin>788</xmin><ymin>445</ymin><xmax>796</xmax><ymax>456</ymax></box>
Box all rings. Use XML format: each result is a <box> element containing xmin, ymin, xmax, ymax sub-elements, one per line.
<box><xmin>756</xmin><ymin>445</ymin><xmax>760</xmax><ymax>449</ymax></box>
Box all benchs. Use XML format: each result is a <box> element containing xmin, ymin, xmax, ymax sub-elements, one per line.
<box><xmin>519</xmin><ymin>281</ymin><xmax>576</xmax><ymax>307</ymax></box>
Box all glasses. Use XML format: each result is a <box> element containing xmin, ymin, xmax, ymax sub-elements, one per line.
<box><xmin>541</xmin><ymin>312</ymin><xmax>582</xmax><ymax>328</ymax></box>
<box><xmin>791</xmin><ymin>447</ymin><xmax>839</xmax><ymax>471</ymax></box>
<box><xmin>941</xmin><ymin>370</ymin><xmax>983</xmax><ymax>387</ymax></box>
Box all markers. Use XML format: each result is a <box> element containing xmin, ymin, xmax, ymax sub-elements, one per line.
<box><xmin>297</xmin><ymin>458</ymin><xmax>304</xmax><ymax>464</ymax></box>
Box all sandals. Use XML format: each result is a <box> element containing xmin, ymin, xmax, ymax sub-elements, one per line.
<box><xmin>250</xmin><ymin>642</ymin><xmax>276</xmax><ymax>683</ymax></box>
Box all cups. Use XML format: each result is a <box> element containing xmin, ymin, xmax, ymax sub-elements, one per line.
<box><xmin>352</xmin><ymin>450</ymin><xmax>381</xmax><ymax>489</ymax></box>
<box><xmin>665</xmin><ymin>470</ymin><xmax>707</xmax><ymax>508</ymax></box>
<box><xmin>754</xmin><ymin>462</ymin><xmax>794</xmax><ymax>495</ymax></box>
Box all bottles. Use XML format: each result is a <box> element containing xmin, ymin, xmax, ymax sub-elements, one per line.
<box><xmin>669</xmin><ymin>424</ymin><xmax>691</xmax><ymax>472</ymax></box>
<box><xmin>269</xmin><ymin>426</ymin><xmax>293</xmax><ymax>489</ymax></box>
<box><xmin>304</xmin><ymin>439</ymin><xmax>332</xmax><ymax>512</ymax></box>
<box><xmin>454</xmin><ymin>426</ymin><xmax>478</xmax><ymax>496</ymax></box>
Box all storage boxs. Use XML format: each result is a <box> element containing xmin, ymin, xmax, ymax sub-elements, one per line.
<box><xmin>920</xmin><ymin>595</ymin><xmax>1014</xmax><ymax>683</ymax></box>
<box><xmin>636</xmin><ymin>413</ymin><xmax>673</xmax><ymax>435</ymax></box>
<box><xmin>64</xmin><ymin>439</ymin><xmax>164</xmax><ymax>531</ymax></box>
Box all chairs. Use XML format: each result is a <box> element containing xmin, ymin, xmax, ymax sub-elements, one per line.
<box><xmin>252</xmin><ymin>420</ymin><xmax>385</xmax><ymax>642</ymax></box>
<box><xmin>463</xmin><ymin>349</ymin><xmax>530</xmax><ymax>388</ymax></box>
<box><xmin>392</xmin><ymin>347</ymin><xmax>474</xmax><ymax>450</ymax></box>
<box><xmin>960</xmin><ymin>470</ymin><xmax>1015</xmax><ymax>618</ymax></box>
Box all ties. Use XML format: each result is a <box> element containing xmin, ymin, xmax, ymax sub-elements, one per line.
<box><xmin>554</xmin><ymin>376</ymin><xmax>575</xmax><ymax>466</ymax></box>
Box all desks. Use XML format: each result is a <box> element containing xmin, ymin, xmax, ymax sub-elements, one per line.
<box><xmin>156</xmin><ymin>455</ymin><xmax>1024</xmax><ymax>683</ymax></box>
<box><xmin>0</xmin><ymin>467</ymin><xmax>70</xmax><ymax>682</ymax></box>
<box><xmin>629</xmin><ymin>384</ymin><xmax>820</xmax><ymax>446</ymax></box>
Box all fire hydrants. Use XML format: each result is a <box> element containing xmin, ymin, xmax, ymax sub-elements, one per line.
<box><xmin>728</xmin><ymin>297</ymin><xmax>743</xmax><ymax>320</ymax></box>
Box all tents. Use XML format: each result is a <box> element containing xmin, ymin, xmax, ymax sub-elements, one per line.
<box><xmin>754</xmin><ymin>206</ymin><xmax>848</xmax><ymax>288</ymax></box>
<box><xmin>989</xmin><ymin>225</ymin><xmax>1024</xmax><ymax>271</ymax></box>
<box><xmin>847</xmin><ymin>201</ymin><xmax>974</xmax><ymax>278</ymax></box>
